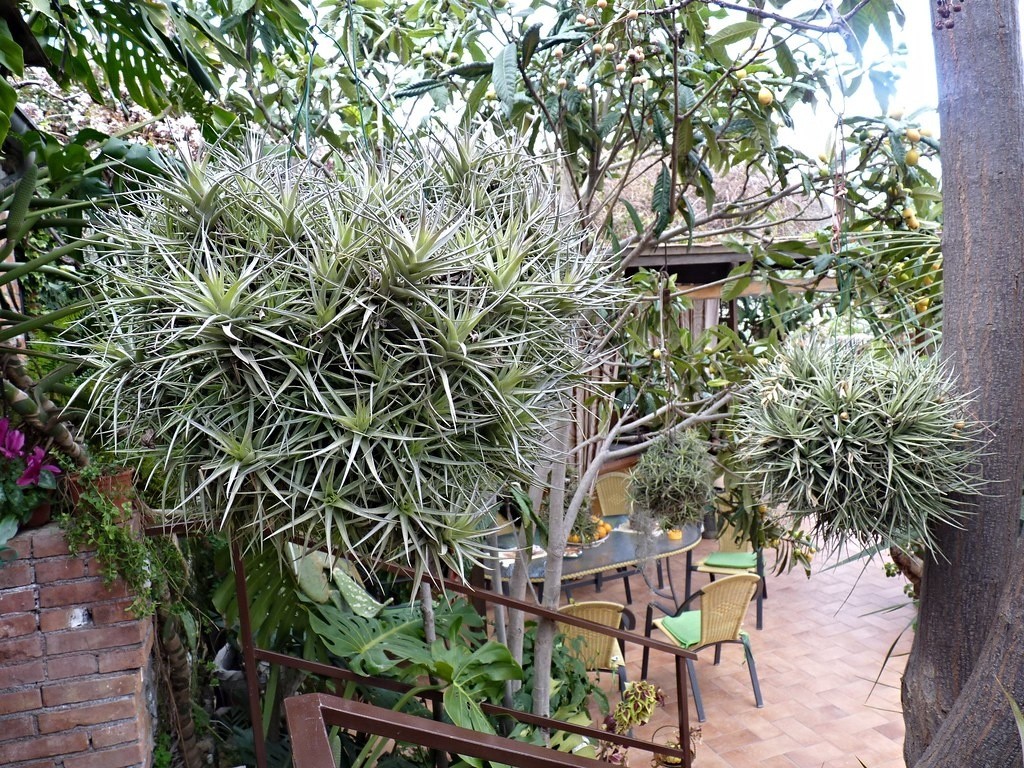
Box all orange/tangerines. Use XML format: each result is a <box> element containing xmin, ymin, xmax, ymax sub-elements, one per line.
<box><xmin>567</xmin><ymin>514</ymin><xmax>611</xmax><ymax>543</ymax></box>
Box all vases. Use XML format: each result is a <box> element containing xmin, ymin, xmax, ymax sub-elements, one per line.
<box><xmin>21</xmin><ymin>489</ymin><xmax>51</xmax><ymax>527</ymax></box>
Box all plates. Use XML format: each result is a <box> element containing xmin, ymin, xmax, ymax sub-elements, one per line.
<box><xmin>563</xmin><ymin>549</ymin><xmax>584</xmax><ymax>559</ymax></box>
<box><xmin>566</xmin><ymin>533</ymin><xmax>609</xmax><ymax>548</ymax></box>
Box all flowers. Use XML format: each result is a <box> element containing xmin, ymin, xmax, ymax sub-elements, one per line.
<box><xmin>0</xmin><ymin>419</ymin><xmax>62</xmax><ymax>547</ymax></box>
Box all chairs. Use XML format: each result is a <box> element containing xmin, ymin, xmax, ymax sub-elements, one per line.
<box><xmin>486</xmin><ymin>472</ymin><xmax>769</xmax><ymax>742</ymax></box>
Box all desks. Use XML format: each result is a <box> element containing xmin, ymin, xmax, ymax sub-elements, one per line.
<box><xmin>484</xmin><ymin>509</ymin><xmax>701</xmax><ymax>615</ymax></box>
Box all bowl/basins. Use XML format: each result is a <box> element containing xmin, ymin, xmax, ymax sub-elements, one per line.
<box><xmin>667</xmin><ymin>530</ymin><xmax>682</xmax><ymax>540</ymax></box>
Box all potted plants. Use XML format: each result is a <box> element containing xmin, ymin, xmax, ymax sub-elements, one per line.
<box><xmin>56</xmin><ymin>450</ymin><xmax>184</xmax><ymax>620</ymax></box>
